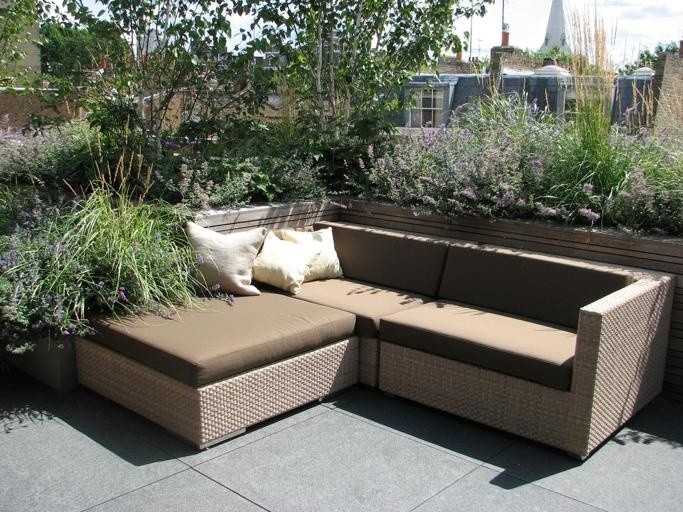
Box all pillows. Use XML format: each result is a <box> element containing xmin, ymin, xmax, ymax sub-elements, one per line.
<box><xmin>178</xmin><ymin>221</ymin><xmax>343</xmax><ymax>297</ymax></box>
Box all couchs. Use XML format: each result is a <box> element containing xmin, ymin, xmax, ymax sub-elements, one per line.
<box><xmin>74</xmin><ymin>218</ymin><xmax>678</xmax><ymax>461</ymax></box>
